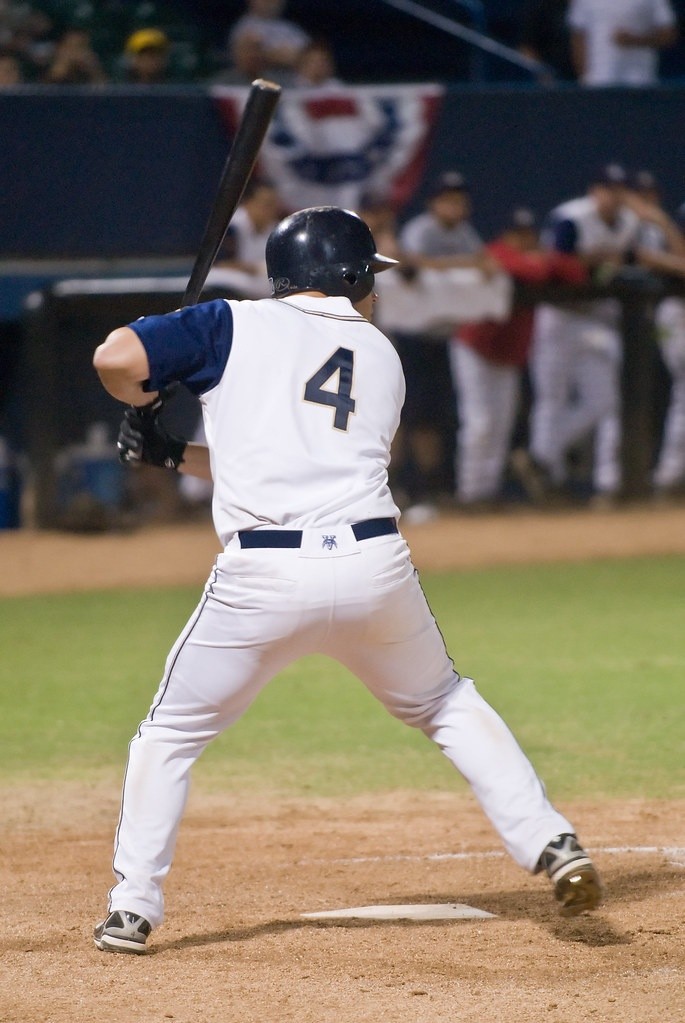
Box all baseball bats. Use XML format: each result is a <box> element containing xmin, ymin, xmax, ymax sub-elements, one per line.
<box><xmin>118</xmin><ymin>77</ymin><xmax>284</xmax><ymax>468</ymax></box>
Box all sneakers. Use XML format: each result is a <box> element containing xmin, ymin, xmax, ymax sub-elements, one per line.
<box><xmin>539</xmin><ymin>834</ymin><xmax>602</xmax><ymax>918</ymax></box>
<box><xmin>90</xmin><ymin>910</ymin><xmax>151</xmax><ymax>954</ymax></box>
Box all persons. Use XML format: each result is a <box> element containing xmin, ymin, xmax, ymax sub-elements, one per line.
<box><xmin>92</xmin><ymin>205</ymin><xmax>602</xmax><ymax>953</ymax></box>
<box><xmin>0</xmin><ymin>0</ymin><xmax>332</xmax><ymax>87</ymax></box>
<box><xmin>565</xmin><ymin>0</ymin><xmax>678</xmax><ymax>89</ymax></box>
<box><xmin>179</xmin><ymin>174</ymin><xmax>685</xmax><ymax>526</ymax></box>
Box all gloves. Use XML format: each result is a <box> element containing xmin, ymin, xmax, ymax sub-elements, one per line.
<box><xmin>118</xmin><ymin>410</ymin><xmax>188</xmax><ymax>471</ymax></box>
<box><xmin>132</xmin><ymin>381</ymin><xmax>181</xmax><ymax>415</ymax></box>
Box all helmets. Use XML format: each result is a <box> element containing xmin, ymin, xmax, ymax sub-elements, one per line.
<box><xmin>264</xmin><ymin>205</ymin><xmax>400</xmax><ymax>304</ymax></box>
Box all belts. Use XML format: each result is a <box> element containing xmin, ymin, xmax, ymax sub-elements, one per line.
<box><xmin>238</xmin><ymin>517</ymin><xmax>398</xmax><ymax>549</ymax></box>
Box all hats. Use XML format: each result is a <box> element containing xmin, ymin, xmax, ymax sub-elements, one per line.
<box><xmin>590</xmin><ymin>156</ymin><xmax>629</xmax><ymax>187</ymax></box>
<box><xmin>126</xmin><ymin>27</ymin><xmax>169</xmax><ymax>55</ymax></box>
<box><xmin>430</xmin><ymin>170</ymin><xmax>469</xmax><ymax>198</ymax></box>
